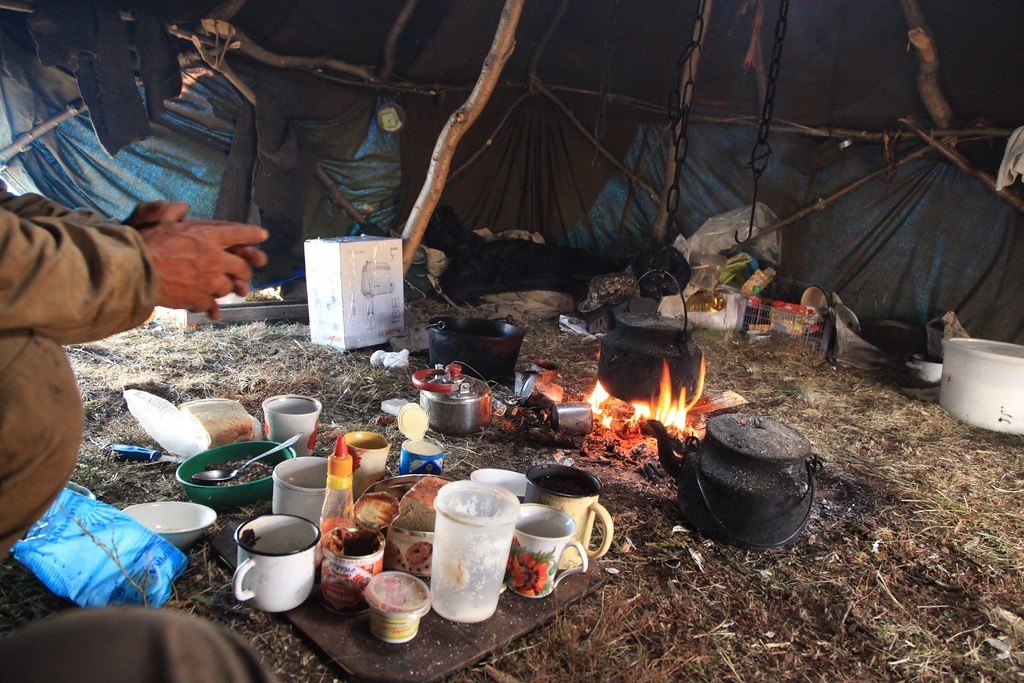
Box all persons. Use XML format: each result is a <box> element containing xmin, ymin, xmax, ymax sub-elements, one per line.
<box><xmin>0</xmin><ymin>179</ymin><xmax>283</xmax><ymax>683</ymax></box>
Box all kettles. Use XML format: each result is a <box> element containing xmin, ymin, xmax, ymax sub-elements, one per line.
<box><xmin>411</xmin><ymin>361</ymin><xmax>493</xmax><ymax>435</ymax></box>
<box><xmin>595</xmin><ymin>266</ymin><xmax>701</xmax><ymax>408</ymax></box>
<box><xmin>645</xmin><ymin>412</ymin><xmax>820</xmax><ymax>549</ymax></box>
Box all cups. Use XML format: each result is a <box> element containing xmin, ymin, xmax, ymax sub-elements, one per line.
<box><xmin>343</xmin><ymin>431</ymin><xmax>391</xmax><ymax>500</ymax></box>
<box><xmin>525</xmin><ymin>464</ymin><xmax>615</xmax><ymax>569</ymax></box>
<box><xmin>261</xmin><ymin>394</ymin><xmax>322</xmax><ymax>457</ymax></box>
<box><xmin>270</xmin><ymin>456</ymin><xmax>336</xmax><ymax>525</ymax></box>
<box><xmin>506</xmin><ymin>503</ymin><xmax>589</xmax><ymax>599</ymax></box>
<box><xmin>468</xmin><ymin>468</ymin><xmax>528</xmax><ymax>498</ymax></box>
<box><xmin>430</xmin><ymin>479</ymin><xmax>522</xmax><ymax>623</ymax></box>
<box><xmin>228</xmin><ymin>513</ymin><xmax>322</xmax><ymax>613</ymax></box>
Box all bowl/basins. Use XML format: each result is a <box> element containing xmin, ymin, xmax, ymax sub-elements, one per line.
<box><xmin>938</xmin><ymin>336</ymin><xmax>1024</xmax><ymax>434</ymax></box>
<box><xmin>176</xmin><ymin>441</ymin><xmax>297</xmax><ymax>508</ymax></box>
<box><xmin>116</xmin><ymin>501</ymin><xmax>218</xmax><ymax>548</ymax></box>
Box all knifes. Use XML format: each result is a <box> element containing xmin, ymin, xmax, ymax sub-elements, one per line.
<box><xmin>110</xmin><ymin>443</ymin><xmax>189</xmax><ymax>466</ymax></box>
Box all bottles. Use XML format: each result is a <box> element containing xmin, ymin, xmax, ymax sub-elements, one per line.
<box><xmin>742</xmin><ymin>285</ymin><xmax>762</xmax><ymax>332</ymax></box>
<box><xmin>319</xmin><ymin>433</ymin><xmax>357</xmax><ymax>535</ymax></box>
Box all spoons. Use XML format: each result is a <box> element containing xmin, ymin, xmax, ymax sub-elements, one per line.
<box><xmin>191</xmin><ymin>429</ymin><xmax>304</xmax><ymax>480</ymax></box>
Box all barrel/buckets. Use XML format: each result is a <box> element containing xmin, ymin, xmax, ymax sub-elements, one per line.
<box><xmin>423</xmin><ymin>313</ymin><xmax>525</xmax><ymax>381</ymax></box>
